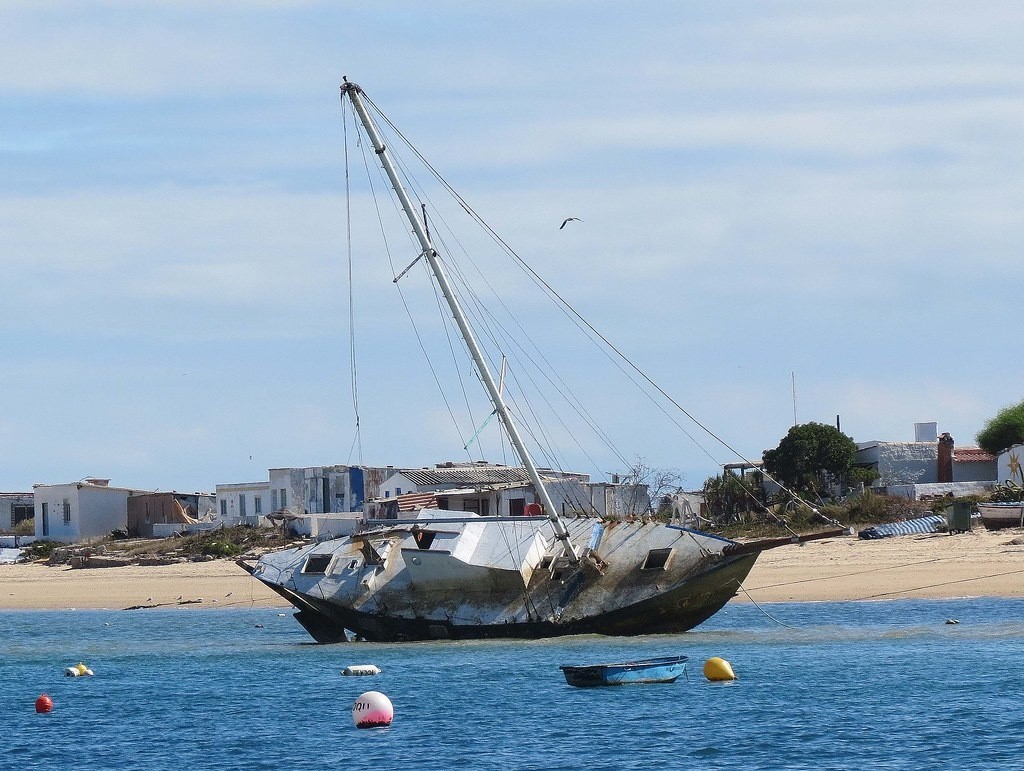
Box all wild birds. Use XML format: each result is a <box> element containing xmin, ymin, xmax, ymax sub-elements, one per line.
<box><xmin>225</xmin><ymin>592</ymin><xmax>233</xmax><ymax>599</ymax></box>
<box><xmin>146</xmin><ymin>596</ymin><xmax>153</xmax><ymax>603</ymax></box>
<box><xmin>559</xmin><ymin>216</ymin><xmax>584</xmax><ymax>230</ymax></box>
<box><xmin>177</xmin><ymin>595</ymin><xmax>183</xmax><ymax>603</ymax></box>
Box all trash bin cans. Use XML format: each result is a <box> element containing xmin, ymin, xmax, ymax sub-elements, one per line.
<box><xmin>945</xmin><ymin>500</ymin><xmax>971</xmax><ymax>535</ymax></box>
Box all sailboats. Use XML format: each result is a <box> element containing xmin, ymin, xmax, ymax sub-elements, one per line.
<box><xmin>231</xmin><ymin>75</ymin><xmax>856</xmax><ymax>642</ymax></box>
